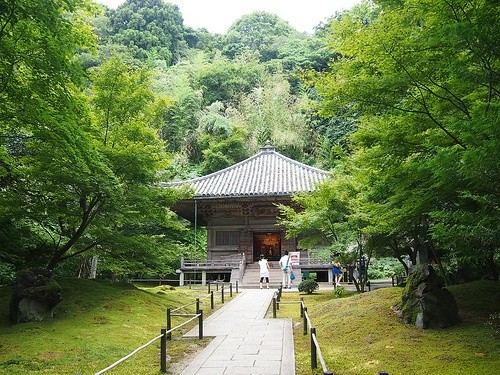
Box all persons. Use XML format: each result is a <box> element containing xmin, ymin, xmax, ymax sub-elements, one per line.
<box><xmin>332</xmin><ymin>256</ymin><xmax>344</xmax><ymax>292</ymax></box>
<box><xmin>279</xmin><ymin>250</ymin><xmax>293</xmax><ymax>288</ymax></box>
<box><xmin>354</xmin><ymin>251</ymin><xmax>370</xmax><ymax>284</ymax></box>
<box><xmin>258</xmin><ymin>254</ymin><xmax>270</xmax><ymax>289</ymax></box>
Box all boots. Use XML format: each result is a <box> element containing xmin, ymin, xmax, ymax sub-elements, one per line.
<box><xmin>259</xmin><ymin>283</ymin><xmax>271</xmax><ymax>289</ymax></box>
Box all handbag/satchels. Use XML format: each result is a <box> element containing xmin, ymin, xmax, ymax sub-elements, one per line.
<box><xmin>290</xmin><ymin>273</ymin><xmax>295</xmax><ymax>280</ymax></box>
<box><xmin>281</xmin><ymin>266</ymin><xmax>287</xmax><ymax>271</ymax></box>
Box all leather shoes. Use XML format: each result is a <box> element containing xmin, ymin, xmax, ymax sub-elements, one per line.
<box><xmin>283</xmin><ymin>286</ymin><xmax>291</xmax><ymax>289</ymax></box>
